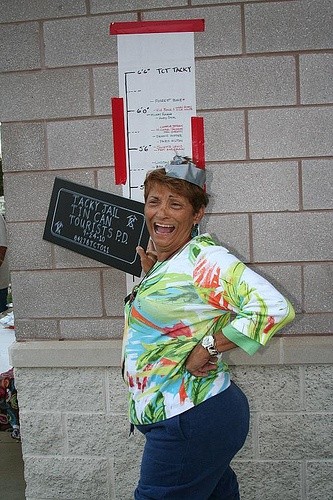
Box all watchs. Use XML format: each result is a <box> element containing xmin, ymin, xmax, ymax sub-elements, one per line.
<box><xmin>202</xmin><ymin>335</ymin><xmax>220</xmax><ymax>357</ymax></box>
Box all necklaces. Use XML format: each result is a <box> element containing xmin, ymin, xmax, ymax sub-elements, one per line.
<box><xmin>130</xmin><ymin>237</ymin><xmax>193</xmax><ymax>306</ymax></box>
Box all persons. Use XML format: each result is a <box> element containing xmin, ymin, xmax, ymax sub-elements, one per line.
<box><xmin>1</xmin><ymin>212</ymin><xmax>10</xmax><ymax>313</ymax></box>
<box><xmin>122</xmin><ymin>163</ymin><xmax>296</xmax><ymax>500</ymax></box>
